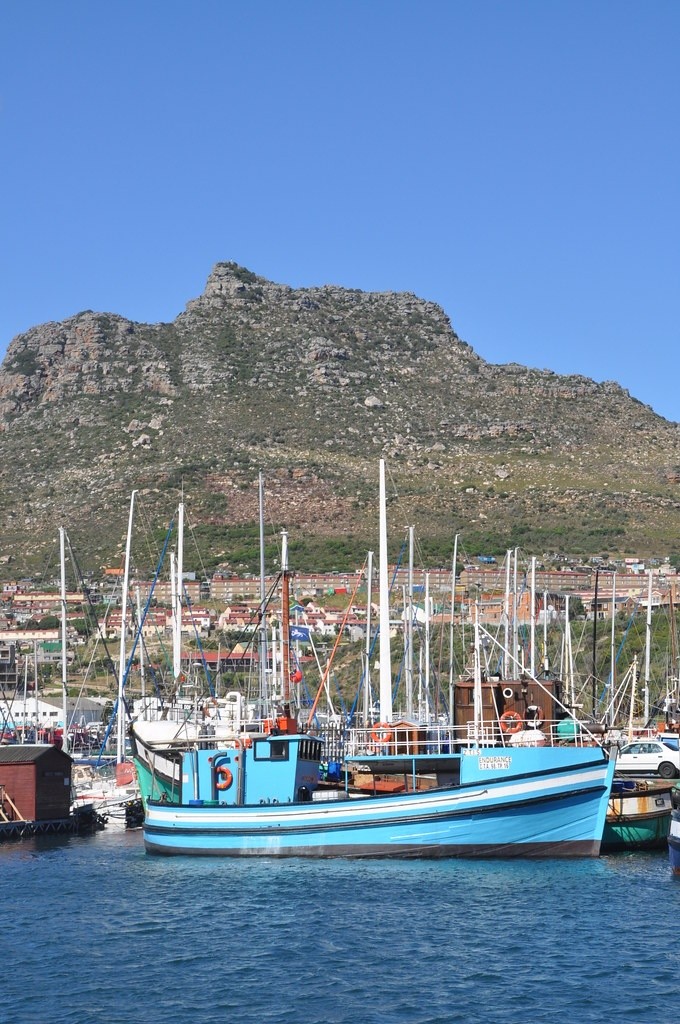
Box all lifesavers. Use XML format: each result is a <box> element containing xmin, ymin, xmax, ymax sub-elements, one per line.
<box><xmin>501</xmin><ymin>711</ymin><xmax>522</xmax><ymax>733</ymax></box>
<box><xmin>291</xmin><ymin>671</ymin><xmax>302</xmax><ymax>683</ymax></box>
<box><xmin>503</xmin><ymin>689</ymin><xmax>513</xmax><ymax>698</ymax></box>
<box><xmin>214</xmin><ymin>766</ymin><xmax>232</xmax><ymax>789</ymax></box>
<box><xmin>370</xmin><ymin>721</ymin><xmax>391</xmax><ymax>743</ymax></box>
<box><xmin>522</xmin><ymin>704</ymin><xmax>544</xmax><ymax>727</ymax></box>
<box><xmin>203</xmin><ymin>696</ymin><xmax>220</xmax><ymax>719</ymax></box>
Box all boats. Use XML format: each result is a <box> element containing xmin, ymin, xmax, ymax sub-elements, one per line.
<box><xmin>129</xmin><ymin>529</ymin><xmax>621</xmax><ymax>861</ymax></box>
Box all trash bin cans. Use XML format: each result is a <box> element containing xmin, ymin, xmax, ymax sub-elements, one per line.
<box><xmin>319</xmin><ymin>762</ymin><xmax>342</xmax><ymax>781</ymax></box>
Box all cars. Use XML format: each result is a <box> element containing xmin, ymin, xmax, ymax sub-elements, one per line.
<box><xmin>614</xmin><ymin>741</ymin><xmax>680</xmax><ymax>778</ymax></box>
<box><xmin>656</xmin><ymin>732</ymin><xmax>679</xmax><ymax>742</ymax></box>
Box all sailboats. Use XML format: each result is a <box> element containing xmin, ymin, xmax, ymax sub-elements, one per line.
<box><xmin>0</xmin><ymin>458</ymin><xmax>680</xmax><ymax>845</ymax></box>
